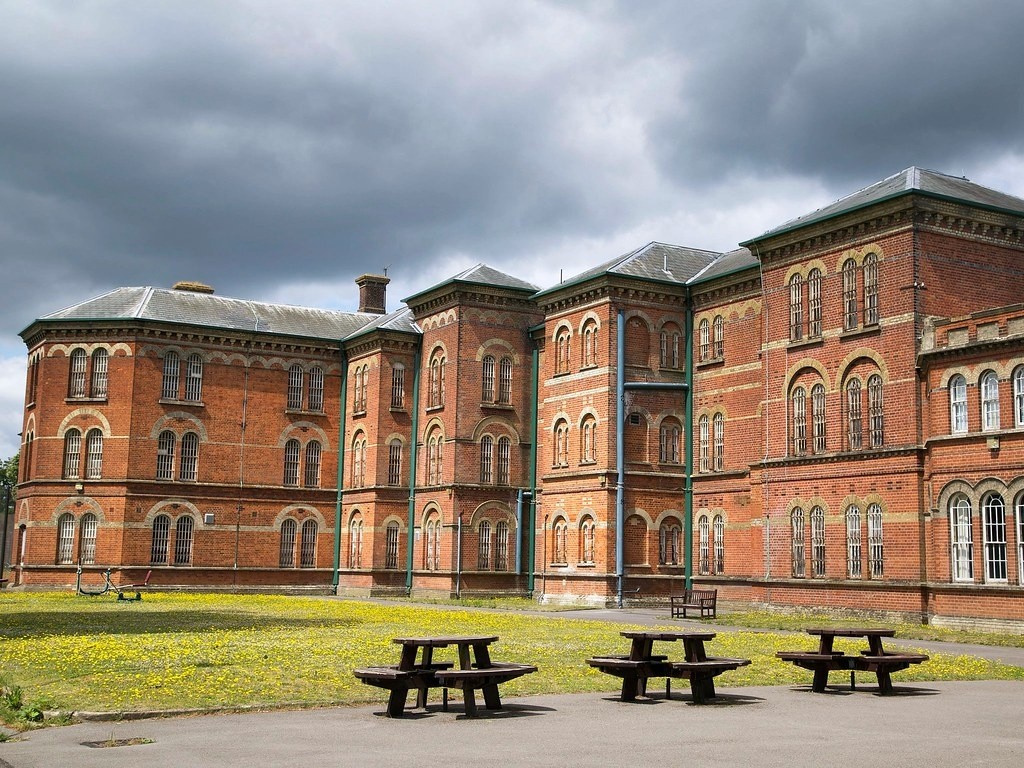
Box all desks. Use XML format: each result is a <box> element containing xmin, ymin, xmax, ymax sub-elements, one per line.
<box><xmin>386</xmin><ymin>635</ymin><xmax>503</xmax><ymax>718</ymax></box>
<box><xmin>619</xmin><ymin>631</ymin><xmax>716</xmax><ymax>703</ymax></box>
<box><xmin>805</xmin><ymin>627</ymin><xmax>896</xmax><ymax>693</ymax></box>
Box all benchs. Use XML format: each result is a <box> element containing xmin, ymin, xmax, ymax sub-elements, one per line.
<box><xmin>584</xmin><ymin>655</ymin><xmax>753</xmax><ymax>678</ymax></box>
<box><xmin>671</xmin><ymin>589</ymin><xmax>718</xmax><ymax>620</ymax></box>
<box><xmin>775</xmin><ymin>650</ymin><xmax>930</xmax><ymax>671</ymax></box>
<box><xmin>353</xmin><ymin>660</ymin><xmax>538</xmax><ymax>689</ymax></box>
